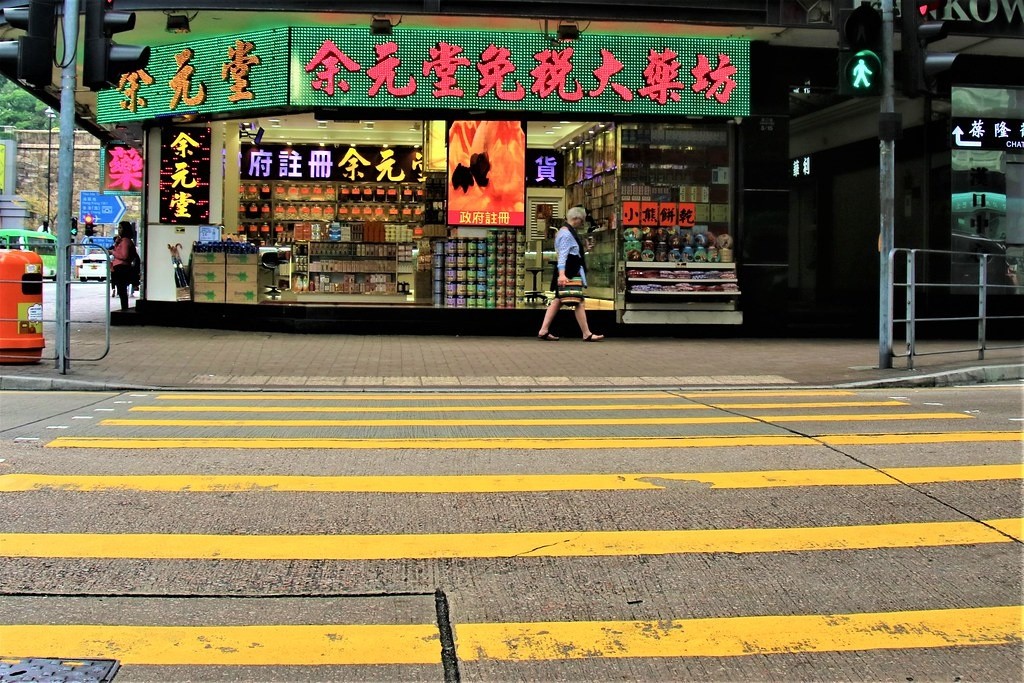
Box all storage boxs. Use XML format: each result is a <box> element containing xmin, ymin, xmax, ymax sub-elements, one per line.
<box><xmin>191</xmin><ymin>250</ymin><xmax>258</xmax><ymax>304</ymax></box>
<box><xmin>308</xmin><ymin>243</ymin><xmax>396</xmax><ymax>291</ymax></box>
<box><xmin>737</xmin><ymin>115</ymin><xmax>790</xmax><ymax>336</ymax></box>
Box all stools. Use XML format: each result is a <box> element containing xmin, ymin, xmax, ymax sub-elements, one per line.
<box><xmin>523</xmin><ymin>267</ymin><xmax>547</xmax><ymax>304</ymax></box>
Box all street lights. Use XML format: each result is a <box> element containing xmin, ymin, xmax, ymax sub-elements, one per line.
<box><xmin>45</xmin><ymin>107</ymin><xmax>56</xmax><ymax>225</ymax></box>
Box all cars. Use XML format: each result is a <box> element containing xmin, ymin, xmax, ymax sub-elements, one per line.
<box><xmin>78</xmin><ymin>253</ymin><xmax>109</xmax><ymax>282</ymax></box>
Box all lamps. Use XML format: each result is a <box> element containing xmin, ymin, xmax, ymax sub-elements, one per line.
<box><xmin>164</xmin><ymin>7</ymin><xmax>193</xmax><ymax>35</ymax></box>
<box><xmin>371</xmin><ymin>11</ymin><xmax>405</xmax><ymax>37</ymax></box>
<box><xmin>557</xmin><ymin>16</ymin><xmax>586</xmax><ymax>41</ymax></box>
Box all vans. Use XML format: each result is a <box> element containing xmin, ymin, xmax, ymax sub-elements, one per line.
<box><xmin>0</xmin><ymin>229</ymin><xmax>58</xmax><ymax>281</ymax></box>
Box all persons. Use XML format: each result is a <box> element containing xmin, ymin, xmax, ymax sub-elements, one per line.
<box><xmin>538</xmin><ymin>207</ymin><xmax>604</xmax><ymax>342</ymax></box>
<box><xmin>108</xmin><ymin>221</ymin><xmax>139</xmax><ymax>311</ymax></box>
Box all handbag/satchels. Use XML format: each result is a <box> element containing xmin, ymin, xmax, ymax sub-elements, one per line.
<box><xmin>558</xmin><ymin>276</ymin><xmax>583</xmax><ymax>307</ymax></box>
<box><xmin>130</xmin><ymin>254</ymin><xmax>138</xmax><ymax>268</ymax></box>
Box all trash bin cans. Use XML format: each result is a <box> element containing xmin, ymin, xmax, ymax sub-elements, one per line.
<box><xmin>0</xmin><ymin>249</ymin><xmax>46</xmax><ymax>364</ymax></box>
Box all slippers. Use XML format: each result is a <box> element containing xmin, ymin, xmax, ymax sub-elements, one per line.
<box><xmin>583</xmin><ymin>333</ymin><xmax>604</xmax><ymax>342</ymax></box>
<box><xmin>538</xmin><ymin>332</ymin><xmax>560</xmax><ymax>341</ymax></box>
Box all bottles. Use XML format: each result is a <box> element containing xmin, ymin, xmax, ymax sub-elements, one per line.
<box><xmin>194</xmin><ymin>238</ymin><xmax>257</xmax><ymax>254</ymax></box>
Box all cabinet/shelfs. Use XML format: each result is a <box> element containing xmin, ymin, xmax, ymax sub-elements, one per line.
<box><xmin>236</xmin><ymin>179</ymin><xmax>429</xmax><ymax>247</ymax></box>
<box><xmin>307</xmin><ymin>241</ymin><xmax>398</xmax><ymax>295</ymax></box>
<box><xmin>621</xmin><ymin>260</ymin><xmax>743</xmax><ymax>325</ymax></box>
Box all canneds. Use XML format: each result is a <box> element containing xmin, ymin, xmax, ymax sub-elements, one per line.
<box><xmin>434</xmin><ymin>230</ymin><xmax>526</xmax><ymax>308</ymax></box>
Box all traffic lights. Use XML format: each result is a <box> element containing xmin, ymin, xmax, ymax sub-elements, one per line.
<box><xmin>82</xmin><ymin>0</ymin><xmax>150</xmax><ymax>92</ymax></box>
<box><xmin>0</xmin><ymin>0</ymin><xmax>60</xmax><ymax>92</ymax></box>
<box><xmin>900</xmin><ymin>1</ymin><xmax>962</xmax><ymax>99</ymax></box>
<box><xmin>71</xmin><ymin>217</ymin><xmax>78</xmax><ymax>236</ymax></box>
<box><xmin>84</xmin><ymin>216</ymin><xmax>94</xmax><ymax>236</ymax></box>
<box><xmin>841</xmin><ymin>5</ymin><xmax>883</xmax><ymax>97</ymax></box>
